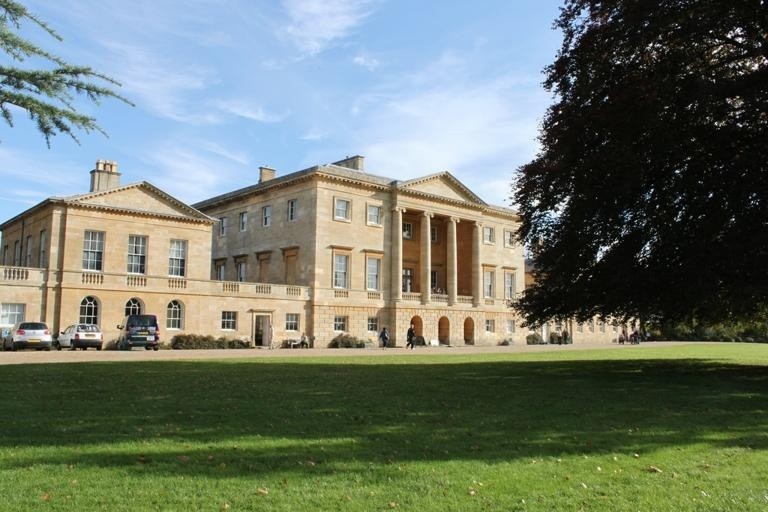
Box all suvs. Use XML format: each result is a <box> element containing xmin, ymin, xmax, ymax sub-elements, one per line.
<box><xmin>116</xmin><ymin>314</ymin><xmax>161</xmax><ymax>350</ymax></box>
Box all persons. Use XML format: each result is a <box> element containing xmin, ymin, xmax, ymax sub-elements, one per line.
<box><xmin>557</xmin><ymin>327</ymin><xmax>562</xmax><ymax>345</ymax></box>
<box><xmin>405</xmin><ymin>324</ymin><xmax>415</xmax><ymax>350</ymax></box>
<box><xmin>268</xmin><ymin>324</ymin><xmax>274</xmax><ymax>349</ymax></box>
<box><xmin>619</xmin><ymin>330</ymin><xmax>639</xmax><ymax>345</ymax></box>
<box><xmin>378</xmin><ymin>326</ymin><xmax>389</xmax><ymax>350</ymax></box>
<box><xmin>301</xmin><ymin>332</ymin><xmax>308</xmax><ymax>349</ymax></box>
<box><xmin>562</xmin><ymin>328</ymin><xmax>569</xmax><ymax>344</ymax></box>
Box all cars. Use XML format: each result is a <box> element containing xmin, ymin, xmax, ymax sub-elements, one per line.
<box><xmin>55</xmin><ymin>323</ymin><xmax>104</xmax><ymax>350</ymax></box>
<box><xmin>2</xmin><ymin>321</ymin><xmax>51</xmax><ymax>351</ymax></box>
